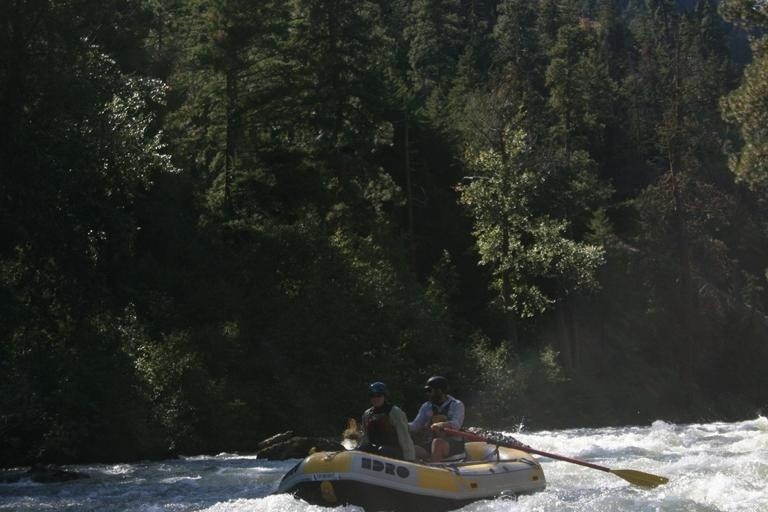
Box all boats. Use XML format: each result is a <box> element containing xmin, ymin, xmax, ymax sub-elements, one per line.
<box><xmin>256</xmin><ymin>428</ymin><xmax>345</xmax><ymax>460</ymax></box>
<box><xmin>277</xmin><ymin>440</ymin><xmax>546</xmax><ymax>512</ymax></box>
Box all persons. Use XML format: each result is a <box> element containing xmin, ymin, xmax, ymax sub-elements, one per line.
<box><xmin>353</xmin><ymin>381</ymin><xmax>416</xmax><ymax>463</ymax></box>
<box><xmin>409</xmin><ymin>375</ymin><xmax>465</xmax><ymax>467</ymax></box>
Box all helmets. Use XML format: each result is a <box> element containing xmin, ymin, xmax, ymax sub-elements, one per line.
<box><xmin>368</xmin><ymin>382</ymin><xmax>386</xmax><ymax>394</ymax></box>
<box><xmin>424</xmin><ymin>376</ymin><xmax>450</xmax><ymax>389</ymax></box>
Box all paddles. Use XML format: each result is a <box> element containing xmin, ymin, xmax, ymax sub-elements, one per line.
<box><xmin>443</xmin><ymin>427</ymin><xmax>669</xmax><ymax>488</ymax></box>
